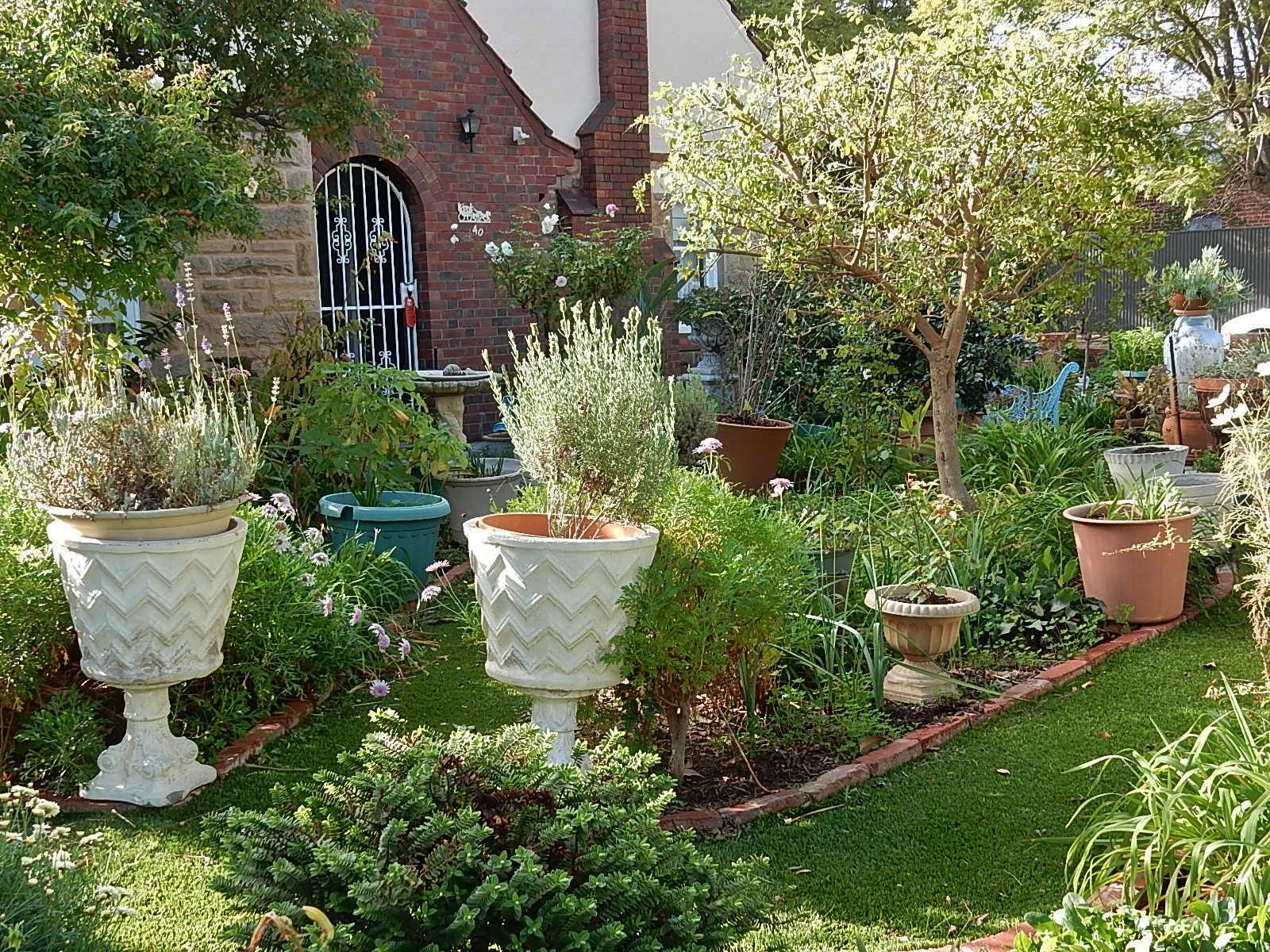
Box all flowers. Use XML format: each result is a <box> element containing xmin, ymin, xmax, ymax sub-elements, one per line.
<box><xmin>449</xmin><ymin>202</ymin><xmax>679</xmax><ymax>537</ymax></box>
<box><xmin>0</xmin><ymin>261</ymin><xmax>282</xmax><ymax>525</ymax></box>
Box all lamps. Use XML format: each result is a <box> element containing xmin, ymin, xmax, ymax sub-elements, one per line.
<box><xmin>458</xmin><ymin>103</ymin><xmax>483</xmax><ymax>153</ymax></box>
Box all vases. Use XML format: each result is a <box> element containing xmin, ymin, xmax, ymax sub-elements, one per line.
<box><xmin>1103</xmin><ymin>444</ymin><xmax>1190</xmax><ymax>501</ymax></box>
<box><xmin>512</xmin><ymin>447</ymin><xmax>578</xmax><ymax>489</ymax></box>
<box><xmin>1145</xmin><ymin>473</ymin><xmax>1236</xmax><ymax>554</ymax></box>
<box><xmin>464</xmin><ymin>512</ymin><xmax>659</xmax><ymax>690</ymax></box>
<box><xmin>481</xmin><ymin>432</ymin><xmax>516</xmax><ymax>459</ymax></box>
<box><xmin>46</xmin><ymin>490</ymin><xmax>253</xmax><ymax>686</ymax></box>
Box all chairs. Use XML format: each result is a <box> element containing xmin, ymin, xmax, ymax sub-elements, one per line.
<box><xmin>982</xmin><ymin>362</ymin><xmax>1079</xmax><ymax>426</ymax></box>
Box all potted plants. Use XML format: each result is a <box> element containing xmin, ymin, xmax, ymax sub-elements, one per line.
<box><xmin>698</xmin><ymin>243</ymin><xmax>1257</xmax><ymax>706</ymax></box>
<box><xmin>276</xmin><ymin>334</ymin><xmax>524</xmax><ymax>604</ymax></box>
